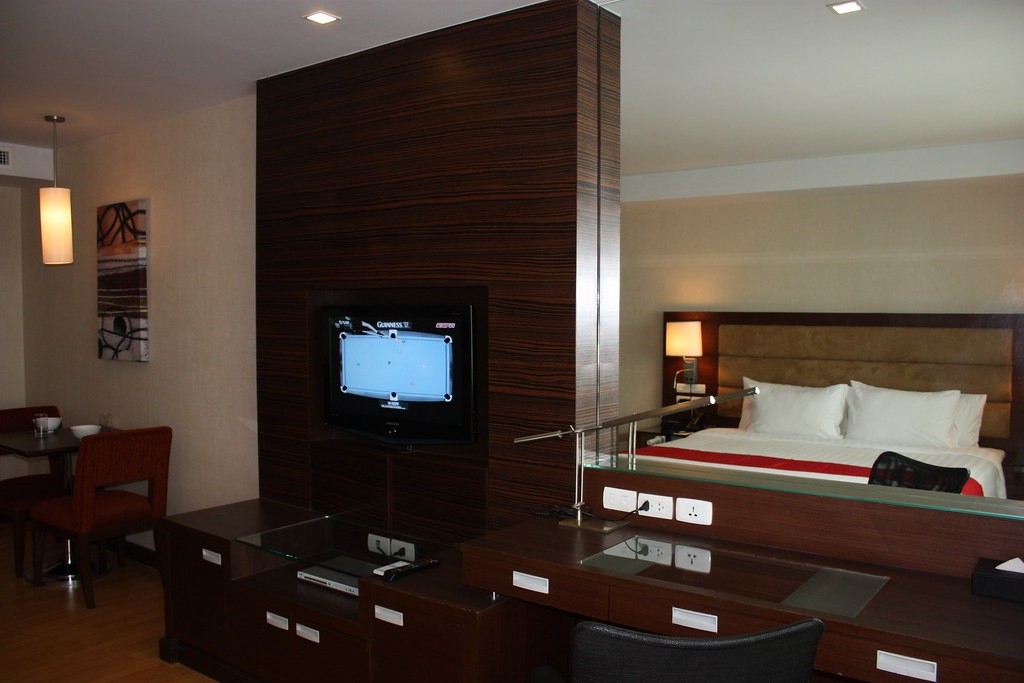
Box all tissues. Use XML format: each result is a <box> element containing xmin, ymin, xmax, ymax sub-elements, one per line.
<box><xmin>972</xmin><ymin>558</ymin><xmax>1024</xmax><ymax>601</ymax></box>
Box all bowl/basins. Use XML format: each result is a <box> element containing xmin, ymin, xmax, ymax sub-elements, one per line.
<box><xmin>71</xmin><ymin>424</ymin><xmax>102</xmax><ymax>442</ymax></box>
<box><xmin>33</xmin><ymin>417</ymin><xmax>62</xmax><ymax>434</ymax></box>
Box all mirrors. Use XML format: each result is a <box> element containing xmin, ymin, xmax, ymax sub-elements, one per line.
<box><xmin>594</xmin><ymin>0</ymin><xmax>1024</xmax><ymax>521</ymax></box>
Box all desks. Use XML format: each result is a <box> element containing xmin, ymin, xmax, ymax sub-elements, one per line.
<box><xmin>459</xmin><ymin>517</ymin><xmax>1024</xmax><ymax>683</ymax></box>
<box><xmin>0</xmin><ymin>425</ymin><xmax>125</xmax><ymax>581</ymax></box>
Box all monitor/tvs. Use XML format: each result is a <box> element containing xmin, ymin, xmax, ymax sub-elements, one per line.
<box><xmin>322</xmin><ymin>304</ymin><xmax>479</xmax><ymax>446</ymax></box>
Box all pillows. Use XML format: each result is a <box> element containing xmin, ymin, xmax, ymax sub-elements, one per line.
<box><xmin>739</xmin><ymin>376</ymin><xmax>987</xmax><ymax>456</ymax></box>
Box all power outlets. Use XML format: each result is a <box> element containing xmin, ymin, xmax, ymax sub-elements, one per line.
<box><xmin>605</xmin><ymin>537</ymin><xmax>711</xmax><ymax>573</ymax></box>
<box><xmin>340</xmin><ymin>329</ymin><xmax>452</xmax><ymax>401</ymax></box>
<box><xmin>366</xmin><ymin>533</ymin><xmax>415</xmax><ymax>561</ymax></box>
<box><xmin>603</xmin><ymin>486</ymin><xmax>713</xmax><ymax>525</ymax></box>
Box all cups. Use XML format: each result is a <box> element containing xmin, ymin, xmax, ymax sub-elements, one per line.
<box><xmin>34</xmin><ymin>413</ymin><xmax>48</xmax><ymax>436</ymax></box>
<box><xmin>99</xmin><ymin>414</ymin><xmax>112</xmax><ymax>434</ymax></box>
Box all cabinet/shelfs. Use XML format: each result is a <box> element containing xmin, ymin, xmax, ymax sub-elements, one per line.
<box><xmin>152</xmin><ymin>500</ymin><xmax>544</xmax><ymax>683</ymax></box>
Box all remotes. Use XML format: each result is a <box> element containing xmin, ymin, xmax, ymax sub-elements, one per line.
<box><xmin>373</xmin><ymin>561</ymin><xmax>411</xmax><ymax>576</ymax></box>
<box><xmin>384</xmin><ymin>559</ymin><xmax>441</xmax><ymax>583</ymax></box>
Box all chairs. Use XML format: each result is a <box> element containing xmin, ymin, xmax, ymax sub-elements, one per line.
<box><xmin>869</xmin><ymin>452</ymin><xmax>972</xmax><ymax>494</ymax></box>
<box><xmin>0</xmin><ymin>404</ymin><xmax>172</xmax><ymax>608</ymax></box>
<box><xmin>559</xmin><ymin>616</ymin><xmax>822</xmax><ymax>682</ymax></box>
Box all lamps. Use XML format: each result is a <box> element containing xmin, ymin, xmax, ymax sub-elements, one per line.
<box><xmin>596</xmin><ymin>386</ymin><xmax>759</xmax><ymax>470</ymax></box>
<box><xmin>39</xmin><ymin>115</ymin><xmax>74</xmax><ymax>265</ymax></box>
<box><xmin>665</xmin><ymin>320</ymin><xmax>702</xmax><ymax>434</ymax></box>
<box><xmin>513</xmin><ymin>395</ymin><xmax>716</xmax><ymax>533</ymax></box>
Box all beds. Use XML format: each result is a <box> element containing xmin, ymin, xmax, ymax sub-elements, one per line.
<box><xmin>618</xmin><ymin>311</ymin><xmax>1024</xmax><ymax>501</ymax></box>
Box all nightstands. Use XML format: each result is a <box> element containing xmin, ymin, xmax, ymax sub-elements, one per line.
<box><xmin>636</xmin><ymin>425</ymin><xmax>702</xmax><ymax>447</ymax></box>
